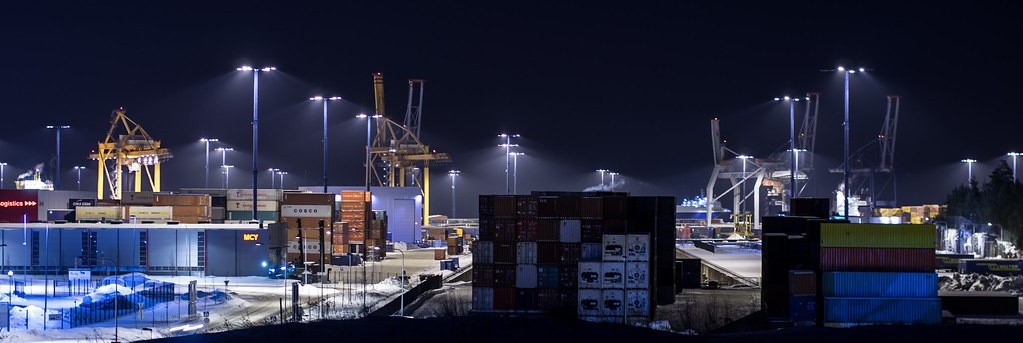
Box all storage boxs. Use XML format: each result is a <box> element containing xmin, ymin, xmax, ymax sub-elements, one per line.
<box><xmin>1</xmin><ymin>184</ymin><xmax>390</xmax><ymax>273</ymax></box>
<box><xmin>471</xmin><ymin>190</ymin><xmax>677</xmax><ymax>325</ymax></box>
<box><xmin>759</xmin><ymin>197</ymin><xmax>1021</xmax><ymax>323</ymax></box>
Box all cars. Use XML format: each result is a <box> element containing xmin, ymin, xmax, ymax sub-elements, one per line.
<box><xmin>100</xmin><ymin>232</ymin><xmax>473</xmax><ymax>313</ymax></box>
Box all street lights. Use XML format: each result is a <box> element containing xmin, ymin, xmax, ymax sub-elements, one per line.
<box><xmin>309</xmin><ymin>95</ymin><xmax>342</xmax><ymax>192</ymax></box>
<box><xmin>776</xmin><ymin>96</ymin><xmax>812</xmax><ymax>197</ymax></box>
<box><xmin>960</xmin><ymin>158</ymin><xmax>978</xmax><ymax>185</ymax></box>
<box><xmin>354</xmin><ymin>112</ymin><xmax>382</xmax><ymax>192</ymax></box>
<box><xmin>236</xmin><ymin>64</ymin><xmax>277</xmax><ymax>221</ymax></box>
<box><xmin>447</xmin><ymin>133</ymin><xmax>619</xmax><ymax>218</ymax></box>
<box><xmin>822</xmin><ymin>64</ymin><xmax>872</xmax><ymax>221</ymax></box>
<box><xmin>1007</xmin><ymin>151</ymin><xmax>1023</xmax><ymax>183</ymax></box>
<box><xmin>48</xmin><ymin>122</ymin><xmax>234</xmax><ymax>194</ymax></box>
